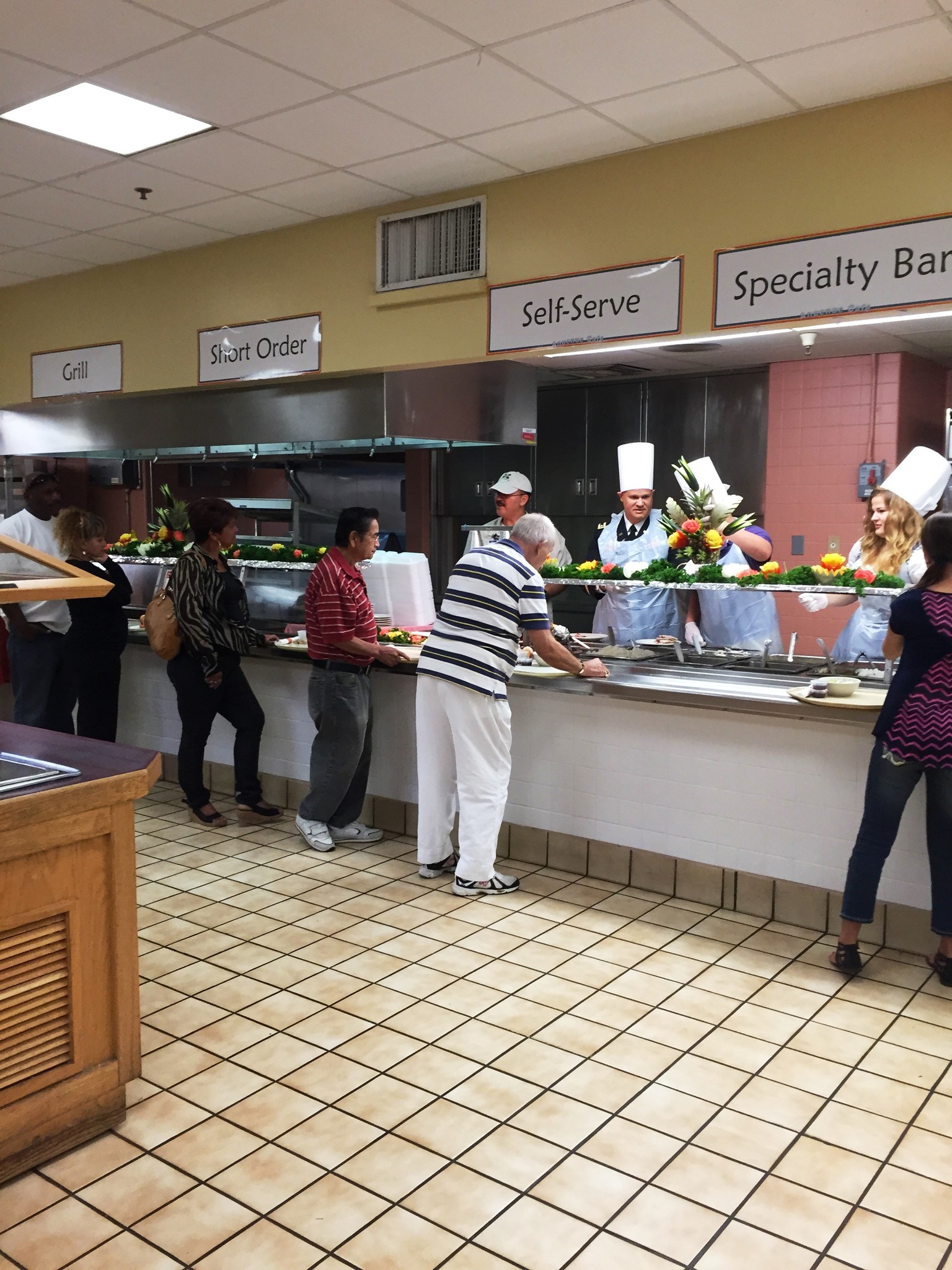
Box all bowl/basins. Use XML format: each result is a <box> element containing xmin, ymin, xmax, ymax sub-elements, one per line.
<box><xmin>810</xmin><ymin>679</ymin><xmax>829</xmax><ymax>699</ymax></box>
<box><xmin>532</xmin><ymin>651</ymin><xmax>551</xmax><ymax>667</ymax></box>
<box><xmin>818</xmin><ymin>676</ymin><xmax>862</xmax><ymax>697</ymax></box>
<box><xmin>516</xmin><ymin>649</ymin><xmax>533</xmax><ymax>666</ymax></box>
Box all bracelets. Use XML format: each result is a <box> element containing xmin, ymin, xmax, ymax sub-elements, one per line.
<box><xmin>573</xmin><ymin>659</ymin><xmax>585</xmax><ymax>675</ymax></box>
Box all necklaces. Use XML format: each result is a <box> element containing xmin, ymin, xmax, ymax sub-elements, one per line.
<box><xmin>68</xmin><ymin>555</ymin><xmax>83</xmax><ymax>561</ymax></box>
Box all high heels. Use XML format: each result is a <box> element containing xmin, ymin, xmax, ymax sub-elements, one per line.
<box><xmin>182</xmin><ymin>799</ymin><xmax>227</xmax><ymax>827</ymax></box>
<box><xmin>236</xmin><ymin>798</ymin><xmax>284</xmax><ymax>828</ymax></box>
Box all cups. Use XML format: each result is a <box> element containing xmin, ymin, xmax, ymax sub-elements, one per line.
<box><xmin>297</xmin><ymin>630</ymin><xmax>308</xmax><ymax>644</ymax></box>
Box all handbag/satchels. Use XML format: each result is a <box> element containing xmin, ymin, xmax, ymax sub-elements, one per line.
<box><xmin>144</xmin><ymin>578</ymin><xmax>181</xmax><ymax>660</ymax></box>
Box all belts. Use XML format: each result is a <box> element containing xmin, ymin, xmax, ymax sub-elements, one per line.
<box><xmin>312</xmin><ymin>659</ymin><xmax>371</xmax><ymax>675</ymax></box>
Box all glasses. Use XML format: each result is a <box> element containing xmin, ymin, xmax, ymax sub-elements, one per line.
<box><xmin>26</xmin><ymin>475</ymin><xmax>56</xmax><ymax>492</ymax></box>
<box><xmin>357</xmin><ymin>532</ymin><xmax>380</xmax><ymax>541</ymax></box>
<box><xmin>495</xmin><ymin>490</ymin><xmax>525</xmax><ymax>500</ymax></box>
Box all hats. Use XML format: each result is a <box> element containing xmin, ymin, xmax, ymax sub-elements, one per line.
<box><xmin>675</xmin><ymin>457</ymin><xmax>729</xmax><ymax>511</ymax></box>
<box><xmin>881</xmin><ymin>447</ymin><xmax>952</xmax><ymax>518</ymax></box>
<box><xmin>488</xmin><ymin>472</ymin><xmax>532</xmax><ymax>495</ymax></box>
<box><xmin>618</xmin><ymin>442</ymin><xmax>654</xmax><ymax>493</ymax></box>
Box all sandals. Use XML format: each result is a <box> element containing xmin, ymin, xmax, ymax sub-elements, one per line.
<box><xmin>926</xmin><ymin>951</ymin><xmax>952</xmax><ymax>988</ymax></box>
<box><xmin>828</xmin><ymin>942</ymin><xmax>862</xmax><ymax>975</ymax></box>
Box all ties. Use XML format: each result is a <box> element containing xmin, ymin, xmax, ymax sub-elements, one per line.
<box><xmin>629</xmin><ymin>524</ymin><xmax>637</xmax><ymax>538</ymax></box>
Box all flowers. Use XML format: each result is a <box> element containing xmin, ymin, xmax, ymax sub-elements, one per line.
<box><xmin>541</xmin><ymin>454</ymin><xmax>907</xmax><ymax>600</ymax></box>
<box><xmin>378</xmin><ymin>625</ymin><xmax>427</xmax><ymax>651</ymax></box>
<box><xmin>99</xmin><ymin>482</ymin><xmax>330</xmax><ymax>563</ymax></box>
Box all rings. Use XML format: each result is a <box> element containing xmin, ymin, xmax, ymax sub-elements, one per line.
<box><xmin>604</xmin><ymin>671</ymin><xmax>608</xmax><ymax>678</ymax></box>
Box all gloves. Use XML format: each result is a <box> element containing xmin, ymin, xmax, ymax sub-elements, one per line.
<box><xmin>605</xmin><ymin>585</ymin><xmax>631</xmax><ymax>596</ymax></box>
<box><xmin>798</xmin><ymin>592</ymin><xmax>828</xmax><ymax>613</ymax></box>
<box><xmin>685</xmin><ymin>621</ymin><xmax>706</xmax><ymax>647</ymax></box>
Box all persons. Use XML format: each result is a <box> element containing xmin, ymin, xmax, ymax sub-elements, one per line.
<box><xmin>0</xmin><ymin>471</ymin><xmax>77</xmax><ymax>735</ymax></box>
<box><xmin>295</xmin><ymin>507</ymin><xmax>411</xmax><ymax>852</ymax></box>
<box><xmin>826</xmin><ymin>513</ymin><xmax>952</xmax><ymax>986</ymax></box>
<box><xmin>51</xmin><ymin>507</ymin><xmax>133</xmax><ymax>743</ymax></box>
<box><xmin>798</xmin><ymin>446</ymin><xmax>952</xmax><ymax>662</ymax></box>
<box><xmin>415</xmin><ymin>512</ymin><xmax>610</xmax><ymax>896</ymax></box>
<box><xmin>164</xmin><ymin>497</ymin><xmax>284</xmax><ymax>827</ymax></box>
<box><xmin>674</xmin><ymin>456</ymin><xmax>785</xmax><ymax>656</ymax></box>
<box><xmin>462</xmin><ymin>472</ymin><xmax>573</xmax><ymax>623</ymax></box>
<box><xmin>579</xmin><ymin>442</ymin><xmax>700</xmax><ymax>648</ymax></box>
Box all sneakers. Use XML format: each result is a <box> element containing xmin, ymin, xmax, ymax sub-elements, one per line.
<box><xmin>452</xmin><ymin>871</ymin><xmax>519</xmax><ymax>896</ymax></box>
<box><xmin>330</xmin><ymin>821</ymin><xmax>384</xmax><ymax>843</ymax></box>
<box><xmin>418</xmin><ymin>850</ymin><xmax>461</xmax><ymax>879</ymax></box>
<box><xmin>295</xmin><ymin>813</ymin><xmax>336</xmax><ymax>852</ymax></box>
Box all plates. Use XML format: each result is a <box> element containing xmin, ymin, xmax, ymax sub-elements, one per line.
<box><xmin>369</xmin><ymin>602</ymin><xmax>392</xmax><ymax>626</ymax></box>
<box><xmin>636</xmin><ymin>638</ymin><xmax>682</xmax><ymax>646</ymax></box>
<box><xmin>570</xmin><ymin>632</ymin><xmax>608</xmax><ymax>642</ymax></box>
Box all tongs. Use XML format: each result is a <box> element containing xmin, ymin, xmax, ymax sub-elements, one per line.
<box><xmin>761</xmin><ymin>639</ymin><xmax>772</xmax><ymax>667</ymax></box>
<box><xmin>787</xmin><ymin>632</ymin><xmax>800</xmax><ymax>662</ymax></box>
<box><xmin>842</xmin><ymin>649</ymin><xmax>875</xmax><ymax>673</ymax></box>
